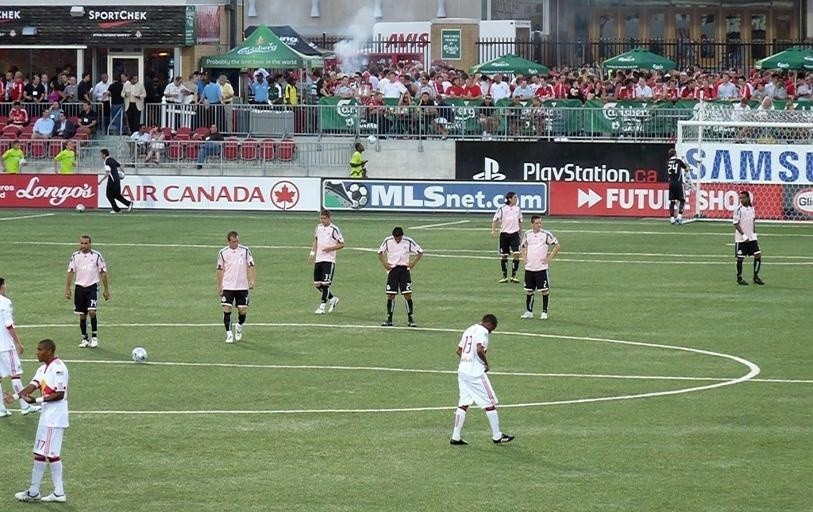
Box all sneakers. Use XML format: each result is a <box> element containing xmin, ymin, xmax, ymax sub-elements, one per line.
<box><xmin>314</xmin><ymin>308</ymin><xmax>326</xmax><ymax>314</ymax></box>
<box><xmin>498</xmin><ymin>277</ymin><xmax>508</xmax><ymax>283</ymax></box>
<box><xmin>91</xmin><ymin>336</ymin><xmax>98</xmax><ymax>349</ymax></box>
<box><xmin>0</xmin><ymin>409</ymin><xmax>12</xmax><ymax>418</ymax></box>
<box><xmin>407</xmin><ymin>320</ymin><xmax>416</xmax><ymax>327</ymax></box>
<box><xmin>509</xmin><ymin>276</ymin><xmax>520</xmax><ymax>283</ymax></box>
<box><xmin>380</xmin><ymin>319</ymin><xmax>395</xmax><ymax>328</ymax></box>
<box><xmin>235</xmin><ymin>322</ymin><xmax>243</xmax><ymax>341</ymax></box>
<box><xmin>449</xmin><ymin>437</ymin><xmax>469</xmax><ymax>446</ymax></box>
<box><xmin>675</xmin><ymin>217</ymin><xmax>683</xmax><ymax>225</ymax></box>
<box><xmin>41</xmin><ymin>490</ymin><xmax>67</xmax><ymax>503</ymax></box>
<box><xmin>540</xmin><ymin>312</ymin><xmax>548</xmax><ymax>322</ymax></box>
<box><xmin>225</xmin><ymin>330</ymin><xmax>234</xmax><ymax>344</ymax></box>
<box><xmin>737</xmin><ymin>277</ymin><xmax>748</xmax><ymax>285</ymax></box>
<box><xmin>752</xmin><ymin>276</ymin><xmax>764</xmax><ymax>287</ymax></box>
<box><xmin>109</xmin><ymin>209</ymin><xmax>123</xmax><ymax>214</ymax></box>
<box><xmin>128</xmin><ymin>201</ymin><xmax>134</xmax><ymax>213</ymax></box>
<box><xmin>196</xmin><ymin>163</ymin><xmax>201</xmax><ymax>168</ymax></box>
<box><xmin>328</xmin><ymin>296</ymin><xmax>340</xmax><ymax>313</ymax></box>
<box><xmin>20</xmin><ymin>403</ymin><xmax>42</xmax><ymax>415</ymax></box>
<box><xmin>519</xmin><ymin>311</ymin><xmax>533</xmax><ymax>319</ymax></box>
<box><xmin>13</xmin><ymin>488</ymin><xmax>42</xmax><ymax>503</ymax></box>
<box><xmin>491</xmin><ymin>433</ymin><xmax>516</xmax><ymax>444</ymax></box>
<box><xmin>78</xmin><ymin>338</ymin><xmax>89</xmax><ymax>351</ymax></box>
<box><xmin>670</xmin><ymin>216</ymin><xmax>675</xmax><ymax>225</ymax></box>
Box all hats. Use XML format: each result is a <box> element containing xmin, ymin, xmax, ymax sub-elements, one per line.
<box><xmin>254</xmin><ymin>70</ymin><xmax>263</xmax><ymax>77</ymax></box>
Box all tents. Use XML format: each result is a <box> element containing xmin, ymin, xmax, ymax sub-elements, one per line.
<box><xmin>200</xmin><ymin>23</ymin><xmax>324</xmax><ymax>107</ymax></box>
<box><xmin>245</xmin><ymin>24</ymin><xmax>335</xmax><ymax>56</ymax></box>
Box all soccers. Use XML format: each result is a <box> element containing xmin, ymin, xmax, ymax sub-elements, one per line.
<box><xmin>348</xmin><ymin>182</ymin><xmax>367</xmax><ymax>208</ymax></box>
<box><xmin>130</xmin><ymin>346</ymin><xmax>149</xmax><ymax>362</ymax></box>
<box><xmin>19</xmin><ymin>159</ymin><xmax>24</xmax><ymax>165</ymax></box>
<box><xmin>117</xmin><ymin>170</ymin><xmax>124</xmax><ymax>179</ymax></box>
<box><xmin>75</xmin><ymin>203</ymin><xmax>85</xmax><ymax>214</ymax></box>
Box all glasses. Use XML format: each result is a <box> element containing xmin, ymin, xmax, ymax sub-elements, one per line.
<box><xmin>403</xmin><ymin>96</ymin><xmax>408</xmax><ymax>99</ymax></box>
<box><xmin>484</xmin><ymin>95</ymin><xmax>491</xmax><ymax>100</ymax></box>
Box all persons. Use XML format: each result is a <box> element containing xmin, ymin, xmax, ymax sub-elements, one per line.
<box><xmin>9</xmin><ymin>71</ymin><xmax>25</xmax><ymax>107</ymax></box>
<box><xmin>733</xmin><ymin>192</ymin><xmax>765</xmax><ymax>285</ymax></box>
<box><xmin>198</xmin><ymin>76</ymin><xmax>224</xmax><ymax>135</ymax></box>
<box><xmin>0</xmin><ymin>80</ymin><xmax>5</xmax><ymax>101</ymax></box>
<box><xmin>120</xmin><ymin>73</ymin><xmax>130</xmax><ymax>86</ymax></box>
<box><xmin>52</xmin><ymin>112</ymin><xmax>75</xmax><ymax>139</ymax></box>
<box><xmin>195</xmin><ymin>70</ymin><xmax>206</xmax><ymax>104</ymax></box>
<box><xmin>23</xmin><ymin>75</ymin><xmax>45</xmax><ymax>116</ymax></box>
<box><xmin>59</xmin><ymin>73</ymin><xmax>69</xmax><ymax>91</ymax></box>
<box><xmin>77</xmin><ymin>72</ymin><xmax>93</xmax><ymax>105</ymax></box>
<box><xmin>202</xmin><ymin>71</ymin><xmax>208</xmax><ymax>85</ymax></box>
<box><xmin>54</xmin><ymin>141</ymin><xmax>85</xmax><ymax>174</ymax></box>
<box><xmin>164</xmin><ymin>76</ymin><xmax>190</xmax><ymax>104</ymax></box>
<box><xmin>76</xmin><ymin>101</ymin><xmax>97</xmax><ymax>133</ymax></box>
<box><xmin>56</xmin><ymin>73</ymin><xmax>62</xmax><ymax>84</ymax></box>
<box><xmin>97</xmin><ymin>149</ymin><xmax>133</xmax><ymax>214</ymax></box>
<box><xmin>520</xmin><ymin>215</ymin><xmax>560</xmax><ymax>319</ymax></box>
<box><xmin>120</xmin><ymin>76</ymin><xmax>146</xmax><ymax>136</ymax></box>
<box><xmin>3</xmin><ymin>72</ymin><xmax>14</xmax><ymax>116</ymax></box>
<box><xmin>2</xmin><ymin>101</ymin><xmax>29</xmax><ymax>131</ymax></box>
<box><xmin>218</xmin><ymin>75</ymin><xmax>234</xmax><ymax>136</ymax></box>
<box><xmin>0</xmin><ymin>277</ymin><xmax>42</xmax><ymax>418</ymax></box>
<box><xmin>183</xmin><ymin>72</ymin><xmax>199</xmax><ymax>105</ymax></box>
<box><xmin>31</xmin><ymin>108</ymin><xmax>55</xmax><ymax>139</ymax></box>
<box><xmin>63</xmin><ymin>76</ymin><xmax>78</xmax><ymax>102</ymax></box>
<box><xmin>667</xmin><ymin>148</ymin><xmax>690</xmax><ymax>225</ymax></box>
<box><xmin>47</xmin><ymin>81</ymin><xmax>66</xmax><ymax>104</ymax></box>
<box><xmin>101</xmin><ymin>75</ymin><xmax>129</xmax><ymax>135</ymax></box>
<box><xmin>449</xmin><ymin>314</ymin><xmax>515</xmax><ymax>445</ymax></box>
<box><xmin>196</xmin><ymin>123</ymin><xmax>224</xmax><ymax>169</ymax></box>
<box><xmin>350</xmin><ymin>143</ymin><xmax>368</xmax><ymax>178</ymax></box>
<box><xmin>41</xmin><ymin>74</ymin><xmax>50</xmax><ymax>110</ymax></box>
<box><xmin>307</xmin><ymin>210</ymin><xmax>345</xmax><ymax>314</ymax></box>
<box><xmin>377</xmin><ymin>227</ymin><xmax>424</xmax><ymax>327</ymax></box>
<box><xmin>1</xmin><ymin>338</ymin><xmax>69</xmax><ymax>503</ymax></box>
<box><xmin>65</xmin><ymin>235</ymin><xmax>112</xmax><ymax>348</ymax></box>
<box><xmin>491</xmin><ymin>192</ymin><xmax>524</xmax><ymax>283</ymax></box>
<box><xmin>49</xmin><ymin>100</ymin><xmax>64</xmax><ymax>121</ymax></box>
<box><xmin>130</xmin><ymin>123</ymin><xmax>149</xmax><ymax>167</ymax></box>
<box><xmin>216</xmin><ymin>231</ymin><xmax>256</xmax><ymax>344</ymax></box>
<box><xmin>144</xmin><ymin>123</ymin><xmax>165</xmax><ymax>164</ymax></box>
<box><xmin>93</xmin><ymin>73</ymin><xmax>111</xmax><ymax>134</ymax></box>
<box><xmin>1</xmin><ymin>140</ymin><xmax>29</xmax><ymax>173</ymax></box>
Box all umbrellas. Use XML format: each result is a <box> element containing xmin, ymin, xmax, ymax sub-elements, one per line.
<box><xmin>603</xmin><ymin>47</ymin><xmax>676</xmax><ymax>70</ymax></box>
<box><xmin>468</xmin><ymin>53</ymin><xmax>549</xmax><ymax>84</ymax></box>
<box><xmin>754</xmin><ymin>48</ymin><xmax>813</xmax><ymax>92</ymax></box>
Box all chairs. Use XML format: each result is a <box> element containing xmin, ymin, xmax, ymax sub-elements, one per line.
<box><xmin>223</xmin><ymin>137</ymin><xmax>295</xmax><ymax>162</ymax></box>
<box><xmin>133</xmin><ymin>127</ymin><xmax>210</xmax><ymax>159</ymax></box>
<box><xmin>0</xmin><ymin>115</ymin><xmax>93</xmax><ymax>161</ymax></box>
